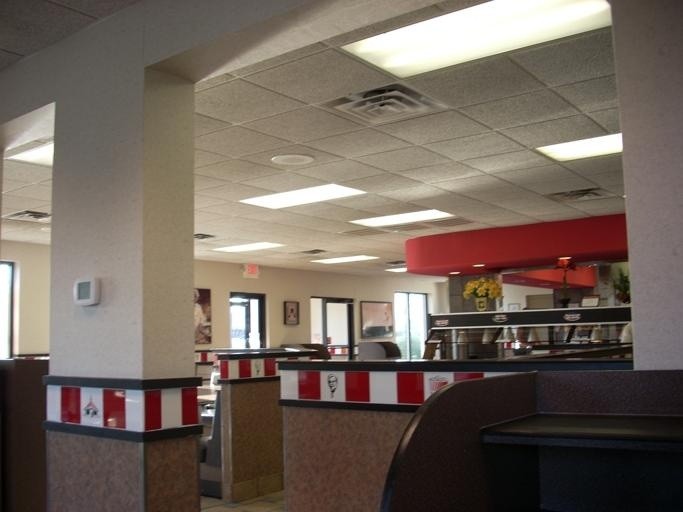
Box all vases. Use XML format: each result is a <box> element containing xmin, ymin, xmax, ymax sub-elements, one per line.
<box><xmin>473</xmin><ymin>299</ymin><xmax>488</xmax><ymax>315</ymax></box>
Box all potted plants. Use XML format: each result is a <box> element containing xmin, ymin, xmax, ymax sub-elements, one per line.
<box><xmin>610</xmin><ymin>261</ymin><xmax>632</xmax><ymax>307</ymax></box>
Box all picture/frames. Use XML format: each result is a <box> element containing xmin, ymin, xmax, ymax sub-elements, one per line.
<box><xmin>283</xmin><ymin>300</ymin><xmax>300</xmax><ymax>325</ymax></box>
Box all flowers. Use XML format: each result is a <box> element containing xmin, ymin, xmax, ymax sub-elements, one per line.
<box><xmin>464</xmin><ymin>275</ymin><xmax>502</xmax><ymax>300</ymax></box>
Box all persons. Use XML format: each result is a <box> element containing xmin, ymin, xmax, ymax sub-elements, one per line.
<box><xmin>321</xmin><ymin>374</ymin><xmax>344</xmax><ymax>401</ymax></box>
